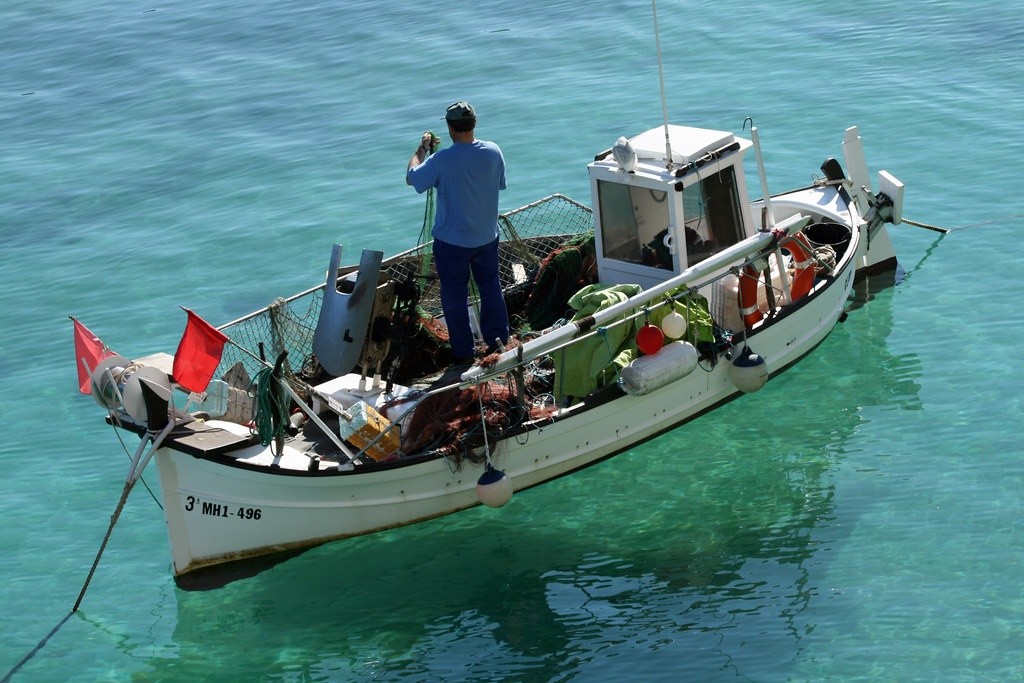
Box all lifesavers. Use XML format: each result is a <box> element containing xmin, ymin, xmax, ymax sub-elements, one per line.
<box><xmin>737</xmin><ymin>233</ymin><xmax>815</xmax><ymax>329</ymax></box>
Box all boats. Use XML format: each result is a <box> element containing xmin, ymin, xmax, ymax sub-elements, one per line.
<box><xmin>89</xmin><ymin>125</ymin><xmax>949</xmax><ymax>593</ymax></box>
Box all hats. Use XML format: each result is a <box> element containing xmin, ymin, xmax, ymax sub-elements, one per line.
<box><xmin>440</xmin><ymin>101</ymin><xmax>477</xmax><ymax>120</ymax></box>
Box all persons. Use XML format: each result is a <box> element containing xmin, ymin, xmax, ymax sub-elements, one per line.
<box><xmin>406</xmin><ymin>102</ymin><xmax>508</xmax><ymax>365</ymax></box>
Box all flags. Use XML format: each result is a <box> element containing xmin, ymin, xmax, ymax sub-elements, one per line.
<box><xmin>171</xmin><ymin>312</ymin><xmax>227</xmax><ymax>393</ymax></box>
<box><xmin>73</xmin><ymin>319</ymin><xmax>108</xmax><ymax>395</ymax></box>
